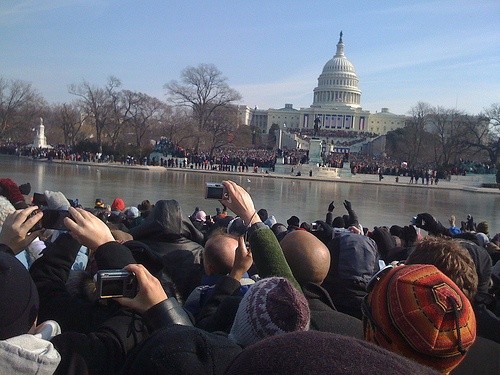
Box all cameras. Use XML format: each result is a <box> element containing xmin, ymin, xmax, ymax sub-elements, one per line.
<box><xmin>42</xmin><ymin>209</ymin><xmax>77</xmax><ymax>231</ymax></box>
<box><xmin>204</xmin><ymin>182</ymin><xmax>229</xmax><ymax>200</ymax></box>
<box><xmin>310</xmin><ymin>223</ymin><xmax>321</xmax><ymax>232</ymax></box>
<box><xmin>93</xmin><ymin>269</ymin><xmax>138</xmax><ymax>298</ymax></box>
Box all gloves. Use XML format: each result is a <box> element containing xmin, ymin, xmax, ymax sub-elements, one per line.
<box><xmin>416</xmin><ymin>211</ymin><xmax>444</xmax><ymax>234</ymax></box>
<box><xmin>343</xmin><ymin>199</ymin><xmax>351</xmax><ymax>210</ymax></box>
<box><xmin>465</xmin><ymin>214</ymin><xmax>473</xmax><ymax>227</ymax></box>
<box><xmin>448</xmin><ymin>214</ymin><xmax>455</xmax><ymax>226</ymax></box>
<box><xmin>328</xmin><ymin>200</ymin><xmax>335</xmax><ymax>211</ymax></box>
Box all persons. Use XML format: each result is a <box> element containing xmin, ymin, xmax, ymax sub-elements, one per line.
<box><xmin>1</xmin><ymin>182</ymin><xmax>500</xmax><ymax>375</ymax></box>
<box><xmin>1</xmin><ymin>112</ymin><xmax>500</xmax><ymax>188</ymax></box>
<box><xmin>312</xmin><ymin>113</ymin><xmax>321</xmax><ymax>135</ymax></box>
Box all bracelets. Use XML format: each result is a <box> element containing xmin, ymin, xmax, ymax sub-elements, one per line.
<box><xmin>244</xmin><ymin>212</ymin><xmax>257</xmax><ymax>228</ymax></box>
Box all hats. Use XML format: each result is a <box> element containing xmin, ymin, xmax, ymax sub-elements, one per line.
<box><xmin>225</xmin><ymin>328</ymin><xmax>438</xmax><ymax>375</ymax></box>
<box><xmin>0</xmin><ymin>251</ymin><xmax>41</xmax><ymax>340</ymax></box>
<box><xmin>126</xmin><ymin>205</ymin><xmax>141</xmax><ymax>218</ymax></box>
<box><xmin>45</xmin><ymin>190</ymin><xmax>71</xmax><ymax>210</ymax></box>
<box><xmin>1</xmin><ymin>178</ymin><xmax>31</xmax><ymax>204</ymax></box>
<box><xmin>111</xmin><ymin>197</ymin><xmax>125</xmax><ymax>210</ymax></box>
<box><xmin>366</xmin><ymin>264</ymin><xmax>477</xmax><ymax>374</ymax></box>
<box><xmin>141</xmin><ymin>199</ymin><xmax>151</xmax><ymax>209</ymax></box>
<box><xmin>371</xmin><ymin>224</ymin><xmax>416</xmax><ymax>250</ymax></box>
<box><xmin>229</xmin><ymin>276</ymin><xmax>310</xmax><ymax>346</ymax></box>
<box><xmin>114</xmin><ymin>325</ymin><xmax>240</xmax><ymax>375</ymax></box>
<box><xmin>332</xmin><ymin>214</ymin><xmax>351</xmax><ymax>228</ymax></box>
<box><xmin>95</xmin><ymin>240</ymin><xmax>136</xmax><ymax>271</ymax></box>
<box><xmin>195</xmin><ymin>210</ymin><xmax>207</xmax><ymax>222</ymax></box>
<box><xmin>258</xmin><ymin>207</ymin><xmax>313</xmax><ymax>236</ymax></box>
